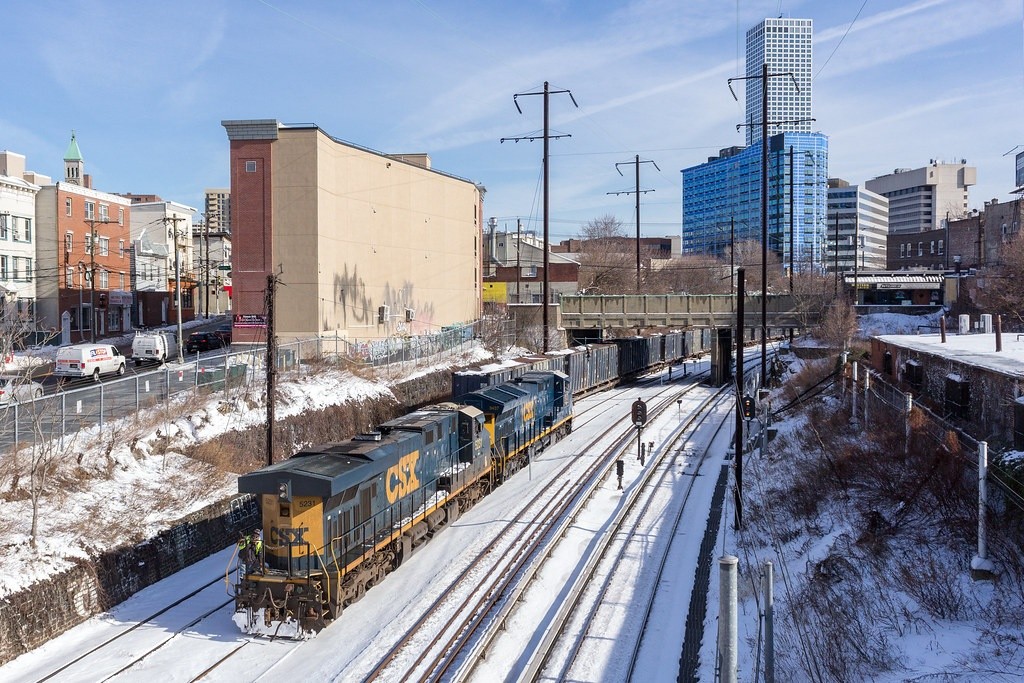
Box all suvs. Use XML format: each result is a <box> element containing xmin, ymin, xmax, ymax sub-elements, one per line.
<box><xmin>185</xmin><ymin>332</ymin><xmax>226</xmax><ymax>354</ymax></box>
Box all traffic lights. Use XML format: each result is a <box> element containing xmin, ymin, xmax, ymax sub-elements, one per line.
<box><xmin>743</xmin><ymin>399</ymin><xmax>756</xmax><ymax>420</ymax></box>
<box><xmin>632</xmin><ymin>401</ymin><xmax>647</xmax><ymax>426</ymax></box>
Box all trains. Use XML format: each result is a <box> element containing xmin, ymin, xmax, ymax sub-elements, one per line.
<box><xmin>223</xmin><ymin>369</ymin><xmax>575</xmax><ymax>644</ymax></box>
<box><xmin>450</xmin><ymin>323</ymin><xmax>821</xmax><ymax>402</ymax></box>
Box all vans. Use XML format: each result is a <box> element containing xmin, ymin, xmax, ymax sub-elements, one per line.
<box><xmin>130</xmin><ymin>328</ymin><xmax>185</xmax><ymax>366</ymax></box>
<box><xmin>52</xmin><ymin>343</ymin><xmax>127</xmax><ymax>382</ymax></box>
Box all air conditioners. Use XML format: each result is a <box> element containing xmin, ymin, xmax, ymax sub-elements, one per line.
<box><xmin>380</xmin><ymin>305</ymin><xmax>390</xmax><ymax>322</ymax></box>
<box><xmin>959</xmin><ymin>315</ymin><xmax>969</xmax><ymax>335</ymax></box>
<box><xmin>406</xmin><ymin>309</ymin><xmax>415</xmax><ymax>321</ymax></box>
<box><xmin>980</xmin><ymin>314</ymin><xmax>992</xmax><ymax>334</ymax></box>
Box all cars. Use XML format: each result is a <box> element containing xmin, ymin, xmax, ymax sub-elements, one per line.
<box><xmin>0</xmin><ymin>375</ymin><xmax>45</xmax><ymax>405</ymax></box>
<box><xmin>215</xmin><ymin>323</ymin><xmax>232</xmax><ymax>338</ymax></box>
